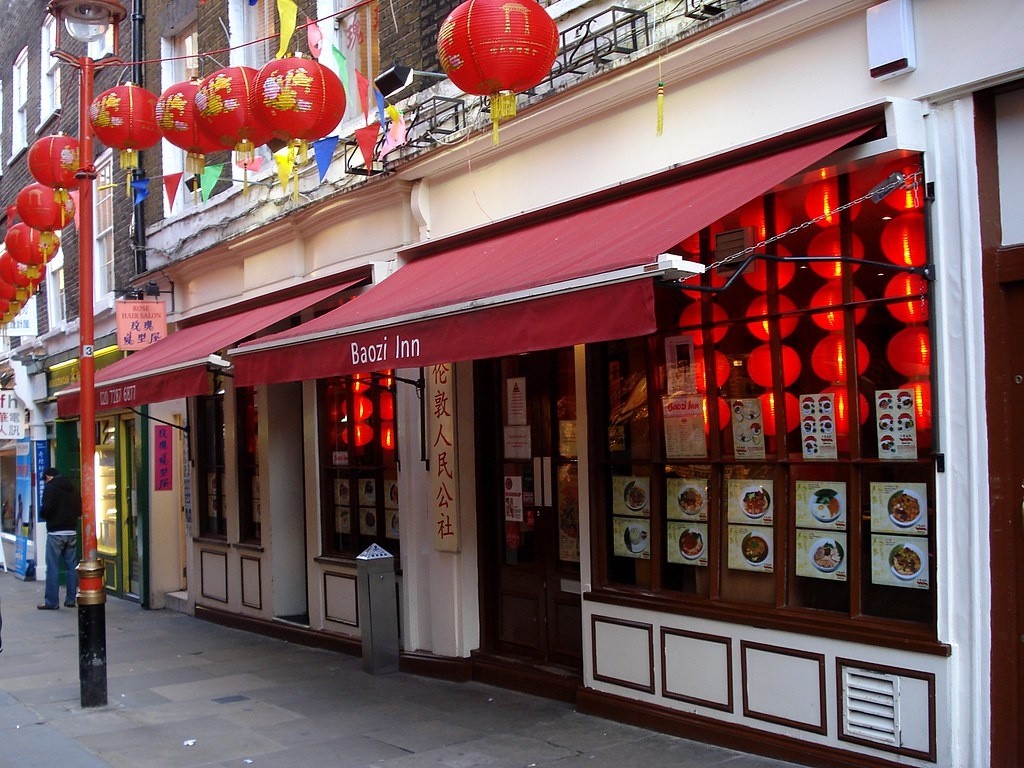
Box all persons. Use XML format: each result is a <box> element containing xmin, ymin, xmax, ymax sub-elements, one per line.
<box><xmin>16</xmin><ymin>494</ymin><xmax>24</xmax><ymax>535</ymax></box>
<box><xmin>36</xmin><ymin>468</ymin><xmax>82</xmax><ymax>610</ymax></box>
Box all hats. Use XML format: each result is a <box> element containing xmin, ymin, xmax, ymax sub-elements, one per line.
<box><xmin>40</xmin><ymin>468</ymin><xmax>59</xmax><ymax>480</ymax></box>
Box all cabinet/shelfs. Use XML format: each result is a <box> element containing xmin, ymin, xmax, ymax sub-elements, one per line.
<box><xmin>100</xmin><ymin>475</ymin><xmax>116</xmax><ymax>525</ymax></box>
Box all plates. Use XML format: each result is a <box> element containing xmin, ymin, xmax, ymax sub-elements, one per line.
<box><xmin>624</xmin><ymin>523</ymin><xmax>648</xmax><ymax>553</ymax></box>
<box><xmin>809</xmin><ymin>538</ymin><xmax>844</xmax><ymax>572</ymax></box>
<box><xmin>740</xmin><ymin>532</ymin><xmax>772</xmax><ymax>567</ymax></box>
<box><xmin>678</xmin><ymin>529</ymin><xmax>705</xmax><ymax>560</ymax></box>
<box><xmin>888</xmin><ymin>543</ymin><xmax>926</xmax><ymax>580</ymax></box>
<box><xmin>734</xmin><ymin>432</ymin><xmax>753</xmax><ymax>442</ymax></box>
<box><xmin>741</xmin><ymin>409</ymin><xmax>760</xmax><ymax>420</ymax></box>
<box><xmin>887</xmin><ymin>489</ymin><xmax>924</xmax><ymax>527</ymax></box>
<box><xmin>624</xmin><ymin>487</ymin><xmax>647</xmax><ymax>511</ymax></box>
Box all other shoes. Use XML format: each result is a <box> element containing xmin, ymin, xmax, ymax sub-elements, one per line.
<box><xmin>64</xmin><ymin>602</ymin><xmax>75</xmax><ymax>607</ymax></box>
<box><xmin>37</xmin><ymin>604</ymin><xmax>59</xmax><ymax>609</ymax></box>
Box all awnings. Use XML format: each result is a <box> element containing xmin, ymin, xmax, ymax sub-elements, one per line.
<box><xmin>52</xmin><ymin>275</ymin><xmax>368</xmax><ymax>419</ymax></box>
<box><xmin>226</xmin><ymin>126</ymin><xmax>875</xmax><ymax>389</ymax></box>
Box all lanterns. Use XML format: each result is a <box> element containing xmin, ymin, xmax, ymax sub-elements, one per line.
<box><xmin>739</xmin><ymin>200</ymin><xmax>802</xmax><ymax>452</ymax></box>
<box><xmin>437</xmin><ymin>0</ymin><xmax>561</xmax><ymax>121</ymax></box>
<box><xmin>249</xmin><ymin>52</ymin><xmax>346</xmax><ymax>164</ymax></box>
<box><xmin>379</xmin><ymin>368</ymin><xmax>396</xmax><ymax>452</ymax></box>
<box><xmin>192</xmin><ymin>66</ymin><xmax>274</xmax><ymax>164</ymax></box>
<box><xmin>0</xmin><ymin>253</ymin><xmax>46</xmax><ymax>329</ymax></box>
<box><xmin>155</xmin><ymin>75</ymin><xmax>227</xmax><ymax>175</ymax></box>
<box><xmin>340</xmin><ymin>372</ymin><xmax>374</xmax><ymax>456</ymax></box>
<box><xmin>89</xmin><ymin>81</ymin><xmax>163</xmax><ymax>170</ymax></box>
<box><xmin>804</xmin><ymin>178</ymin><xmax>869</xmax><ymax>434</ymax></box>
<box><xmin>5</xmin><ymin>221</ymin><xmax>60</xmax><ymax>278</ymax></box>
<box><xmin>15</xmin><ymin>181</ymin><xmax>76</xmax><ymax>235</ymax></box>
<box><xmin>678</xmin><ymin>220</ymin><xmax>731</xmax><ymax>447</ymax></box>
<box><xmin>878</xmin><ymin>164</ymin><xmax>933</xmax><ymax>431</ymax></box>
<box><xmin>27</xmin><ymin>131</ymin><xmax>80</xmax><ymax>203</ymax></box>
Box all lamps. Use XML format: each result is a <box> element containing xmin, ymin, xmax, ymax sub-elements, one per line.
<box><xmin>183</xmin><ymin>173</ymin><xmax>269</xmax><ymax>196</ymax></box>
<box><xmin>123</xmin><ymin>280</ymin><xmax>175</xmax><ymax>302</ymax></box>
<box><xmin>372</xmin><ymin>65</ymin><xmax>448</xmax><ymax>100</ymax></box>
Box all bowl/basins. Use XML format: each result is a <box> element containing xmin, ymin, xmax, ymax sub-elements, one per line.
<box><xmin>809</xmin><ymin>488</ymin><xmax>843</xmax><ymax>523</ymax></box>
<box><xmin>739</xmin><ymin>487</ymin><xmax>770</xmax><ymax>518</ymax></box>
<box><xmin>678</xmin><ymin>485</ymin><xmax>705</xmax><ymax>516</ymax></box>
